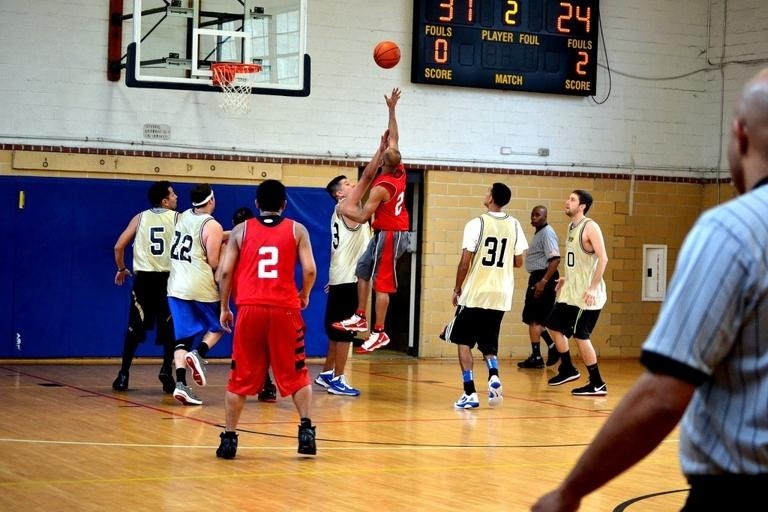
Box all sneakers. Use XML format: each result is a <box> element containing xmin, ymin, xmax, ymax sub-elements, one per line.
<box><xmin>571</xmin><ymin>382</ymin><xmax>607</xmax><ymax>396</ymax></box>
<box><xmin>326</xmin><ymin>375</ymin><xmax>360</xmax><ymax>396</ymax></box>
<box><xmin>112</xmin><ymin>373</ymin><xmax>129</xmax><ymax>392</ymax></box>
<box><xmin>332</xmin><ymin>313</ymin><xmax>370</xmax><ymax>333</ymax></box>
<box><xmin>547</xmin><ymin>364</ymin><xmax>581</xmax><ymax>386</ymax></box>
<box><xmin>173</xmin><ymin>381</ymin><xmax>204</xmax><ymax>406</ymax></box>
<box><xmin>488</xmin><ymin>374</ymin><xmax>503</xmax><ymax>407</ymax></box>
<box><xmin>354</xmin><ymin>330</ymin><xmax>391</xmax><ymax>355</ymax></box>
<box><xmin>517</xmin><ymin>355</ymin><xmax>545</xmax><ymax>369</ymax></box>
<box><xmin>453</xmin><ymin>391</ymin><xmax>480</xmax><ymax>410</ymax></box>
<box><xmin>314</xmin><ymin>368</ymin><xmax>335</xmax><ymax>387</ymax></box>
<box><xmin>258</xmin><ymin>385</ymin><xmax>277</xmax><ymax>402</ymax></box>
<box><xmin>216</xmin><ymin>432</ymin><xmax>238</xmax><ymax>459</ymax></box>
<box><xmin>545</xmin><ymin>344</ymin><xmax>560</xmax><ymax>367</ymax></box>
<box><xmin>184</xmin><ymin>348</ymin><xmax>209</xmax><ymax>388</ymax></box>
<box><xmin>158</xmin><ymin>366</ymin><xmax>176</xmax><ymax>394</ymax></box>
<box><xmin>298</xmin><ymin>425</ymin><xmax>318</xmax><ymax>455</ymax></box>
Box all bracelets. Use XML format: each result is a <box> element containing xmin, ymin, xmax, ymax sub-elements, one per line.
<box><xmin>540</xmin><ymin>277</ymin><xmax>548</xmax><ymax>283</ymax></box>
<box><xmin>118</xmin><ymin>267</ymin><xmax>126</xmax><ymax>272</ymax></box>
<box><xmin>220</xmin><ymin>310</ymin><xmax>232</xmax><ymax>314</ymax></box>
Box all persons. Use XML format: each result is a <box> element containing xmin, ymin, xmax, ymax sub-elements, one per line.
<box><xmin>166</xmin><ymin>182</ymin><xmax>232</xmax><ymax>405</ymax></box>
<box><xmin>216</xmin><ymin>179</ymin><xmax>317</xmax><ymax>460</ymax></box>
<box><xmin>518</xmin><ymin>205</ymin><xmax>562</xmax><ymax>370</ymax></box>
<box><xmin>215</xmin><ymin>207</ymin><xmax>277</xmax><ymax>404</ymax></box>
<box><xmin>314</xmin><ymin>129</ymin><xmax>390</xmax><ymax>397</ymax></box>
<box><xmin>332</xmin><ymin>87</ymin><xmax>412</xmax><ymax>353</ymax></box>
<box><xmin>439</xmin><ymin>182</ymin><xmax>530</xmax><ymax>410</ymax></box>
<box><xmin>547</xmin><ymin>188</ymin><xmax>607</xmax><ymax>396</ymax></box>
<box><xmin>533</xmin><ymin>69</ymin><xmax>767</xmax><ymax>511</ymax></box>
<box><xmin>112</xmin><ymin>181</ymin><xmax>182</xmax><ymax>393</ymax></box>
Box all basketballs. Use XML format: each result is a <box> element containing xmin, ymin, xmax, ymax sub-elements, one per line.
<box><xmin>373</xmin><ymin>41</ymin><xmax>401</xmax><ymax>69</ymax></box>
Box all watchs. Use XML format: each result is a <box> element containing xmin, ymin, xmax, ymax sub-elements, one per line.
<box><xmin>453</xmin><ymin>288</ymin><xmax>461</xmax><ymax>294</ymax></box>
